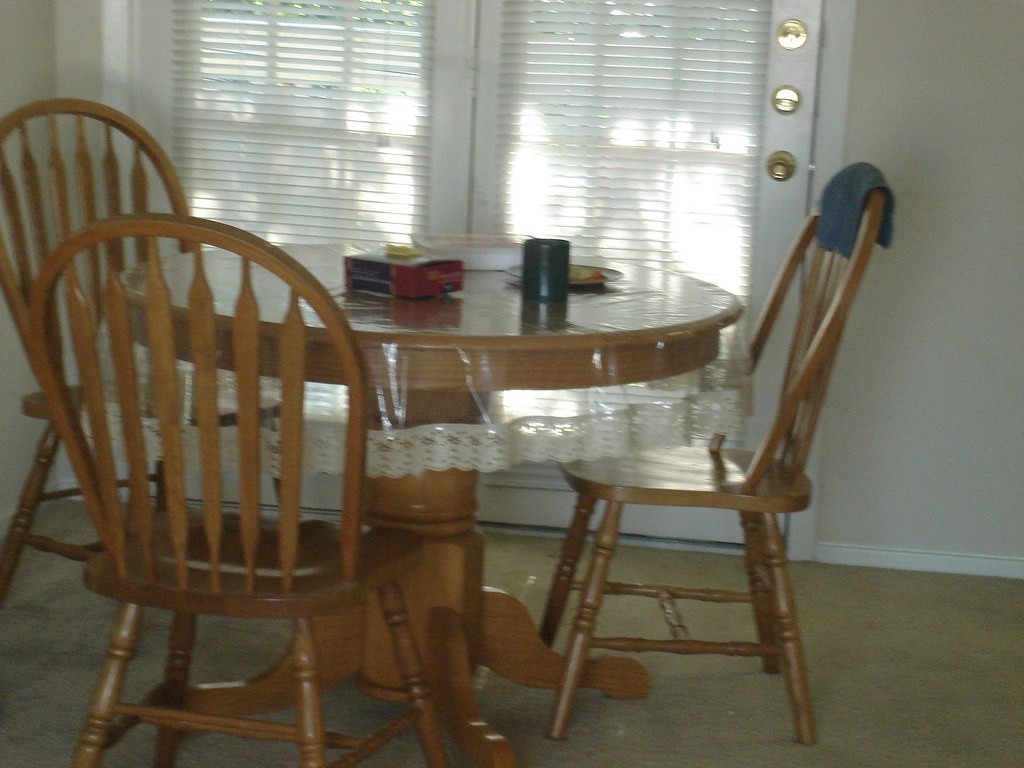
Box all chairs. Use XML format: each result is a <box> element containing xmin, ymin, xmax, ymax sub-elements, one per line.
<box><xmin>534</xmin><ymin>159</ymin><xmax>901</xmax><ymax>743</ymax></box>
<box><xmin>0</xmin><ymin>95</ymin><xmax>296</xmax><ymax>587</ymax></box>
<box><xmin>29</xmin><ymin>216</ymin><xmax>438</xmax><ymax>768</ymax></box>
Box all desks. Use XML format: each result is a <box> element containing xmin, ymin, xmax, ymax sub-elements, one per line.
<box><xmin>103</xmin><ymin>238</ymin><xmax>742</xmax><ymax>767</ymax></box>
<box><xmin>109</xmin><ymin>241</ymin><xmax>745</xmax><ymax>767</ymax></box>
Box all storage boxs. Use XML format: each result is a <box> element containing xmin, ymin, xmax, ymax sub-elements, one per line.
<box><xmin>344</xmin><ymin>251</ymin><xmax>465</xmax><ymax>300</ymax></box>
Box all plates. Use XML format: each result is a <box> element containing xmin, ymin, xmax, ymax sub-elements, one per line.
<box><xmin>505</xmin><ymin>262</ymin><xmax>625</xmax><ymax>289</ymax></box>
